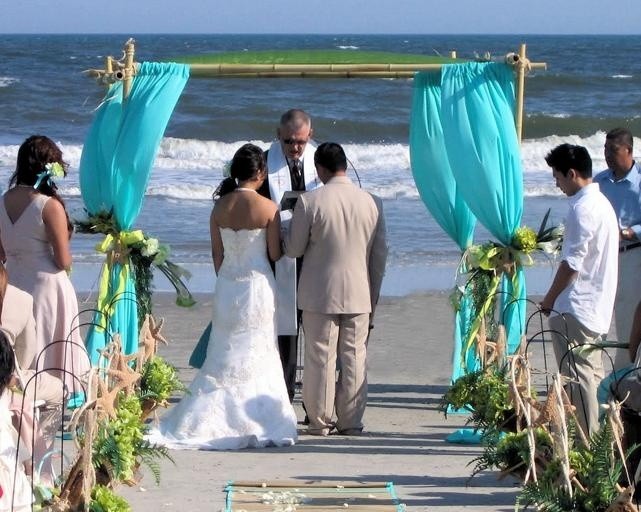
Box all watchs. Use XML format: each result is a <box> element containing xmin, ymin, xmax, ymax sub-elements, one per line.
<box><xmin>621</xmin><ymin>229</ymin><xmax>628</xmax><ymax>240</ymax></box>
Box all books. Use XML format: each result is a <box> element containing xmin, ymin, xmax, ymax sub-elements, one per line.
<box><xmin>278</xmin><ymin>189</ymin><xmax>302</xmax><ymax>211</ymax></box>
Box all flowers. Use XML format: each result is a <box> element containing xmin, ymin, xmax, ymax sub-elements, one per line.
<box><xmin>438</xmin><ymin>207</ymin><xmax>565</xmax><ymax>368</ymax></box>
<box><xmin>67</xmin><ymin>203</ymin><xmax>195</xmax><ymax>308</ymax></box>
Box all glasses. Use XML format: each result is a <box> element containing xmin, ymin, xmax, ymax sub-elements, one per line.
<box><xmin>284</xmin><ymin>139</ymin><xmax>307</xmax><ymax>145</ymax></box>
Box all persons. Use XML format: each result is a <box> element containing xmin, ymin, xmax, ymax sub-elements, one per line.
<box><xmin>281</xmin><ymin>142</ymin><xmax>388</xmax><ymax>435</ymax></box>
<box><xmin>591</xmin><ymin>128</ymin><xmax>641</xmax><ymax>367</ymax></box>
<box><xmin>1</xmin><ymin>246</ymin><xmax>68</xmax><ymax>482</ymax></box>
<box><xmin>0</xmin><ymin>137</ymin><xmax>90</xmax><ymax>395</ymax></box>
<box><xmin>597</xmin><ymin>300</ymin><xmax>641</xmax><ymax>484</ymax></box>
<box><xmin>0</xmin><ymin>332</ymin><xmax>34</xmax><ymax>512</ymax></box>
<box><xmin>256</xmin><ymin>108</ymin><xmax>344</xmax><ymax>405</ymax></box>
<box><xmin>538</xmin><ymin>144</ymin><xmax>620</xmax><ymax>448</ymax></box>
<box><xmin>201</xmin><ymin>143</ymin><xmax>297</xmax><ymax>450</ymax></box>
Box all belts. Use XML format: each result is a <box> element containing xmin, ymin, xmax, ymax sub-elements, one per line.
<box><xmin>619</xmin><ymin>241</ymin><xmax>641</xmax><ymax>259</ymax></box>
